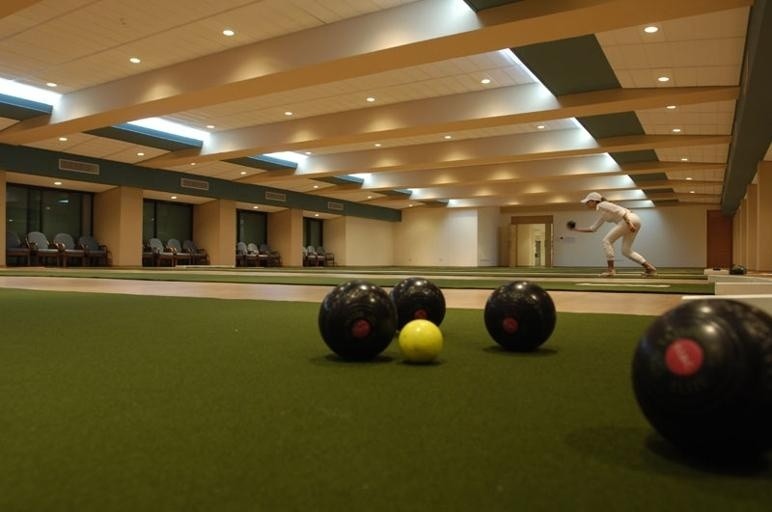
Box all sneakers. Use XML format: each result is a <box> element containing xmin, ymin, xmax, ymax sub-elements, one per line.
<box><xmin>601</xmin><ymin>268</ymin><xmax>657</xmax><ymax>278</ymax></box>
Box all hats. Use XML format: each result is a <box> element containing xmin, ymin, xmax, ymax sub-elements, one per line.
<box><xmin>580</xmin><ymin>191</ymin><xmax>603</xmax><ymax>203</ymax></box>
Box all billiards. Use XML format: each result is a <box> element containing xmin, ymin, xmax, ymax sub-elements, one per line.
<box><xmin>398</xmin><ymin>318</ymin><xmax>443</xmax><ymax>364</ymax></box>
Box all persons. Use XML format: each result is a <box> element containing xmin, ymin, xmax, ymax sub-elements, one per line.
<box><xmin>565</xmin><ymin>192</ymin><xmax>659</xmax><ymax>276</ymax></box>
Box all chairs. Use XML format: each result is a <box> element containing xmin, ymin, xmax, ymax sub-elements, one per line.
<box><xmin>6</xmin><ymin>231</ymin><xmax>110</xmax><ymax>267</ymax></box>
<box><xmin>303</xmin><ymin>246</ymin><xmax>335</xmax><ymax>266</ymax></box>
<box><xmin>236</xmin><ymin>241</ymin><xmax>284</xmax><ymax>267</ymax></box>
<box><xmin>143</xmin><ymin>237</ymin><xmax>212</xmax><ymax>266</ymax></box>
